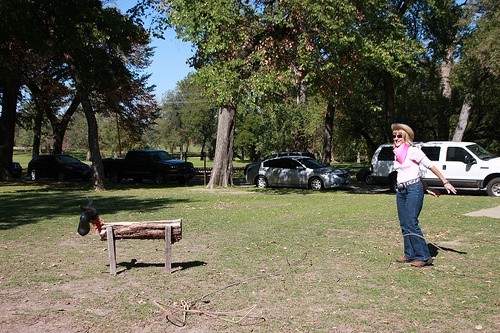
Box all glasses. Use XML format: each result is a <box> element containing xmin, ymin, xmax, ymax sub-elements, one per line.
<box><xmin>393</xmin><ymin>135</ymin><xmax>405</xmax><ymax>138</ymax></box>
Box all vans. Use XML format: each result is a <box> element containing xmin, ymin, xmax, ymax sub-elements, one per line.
<box><xmin>367</xmin><ymin>140</ymin><xmax>500</xmax><ymax>197</ymax></box>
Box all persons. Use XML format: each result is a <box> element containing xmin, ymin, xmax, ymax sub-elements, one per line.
<box><xmin>391</xmin><ymin>123</ymin><xmax>457</xmax><ymax>267</ymax></box>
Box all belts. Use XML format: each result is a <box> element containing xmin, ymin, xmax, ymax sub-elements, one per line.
<box><xmin>397</xmin><ymin>178</ymin><xmax>420</xmax><ymax>189</ymax></box>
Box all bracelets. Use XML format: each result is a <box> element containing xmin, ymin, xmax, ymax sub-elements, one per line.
<box><xmin>443</xmin><ymin>181</ymin><xmax>449</xmax><ymax>185</ymax></box>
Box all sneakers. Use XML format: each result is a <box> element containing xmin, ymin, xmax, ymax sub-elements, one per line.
<box><xmin>396</xmin><ymin>257</ymin><xmax>411</xmax><ymax>262</ymax></box>
<box><xmin>411</xmin><ymin>258</ymin><xmax>432</xmax><ymax>267</ymax></box>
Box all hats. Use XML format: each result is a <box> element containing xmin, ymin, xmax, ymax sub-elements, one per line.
<box><xmin>391</xmin><ymin>123</ymin><xmax>414</xmax><ymax>139</ymax></box>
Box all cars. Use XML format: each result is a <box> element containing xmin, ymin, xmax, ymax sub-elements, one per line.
<box><xmin>27</xmin><ymin>153</ymin><xmax>92</xmax><ymax>182</ymax></box>
<box><xmin>246</xmin><ymin>155</ymin><xmax>350</xmax><ymax>191</ymax></box>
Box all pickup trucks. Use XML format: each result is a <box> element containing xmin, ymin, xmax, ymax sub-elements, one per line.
<box><xmin>101</xmin><ymin>149</ymin><xmax>196</xmax><ymax>186</ymax></box>
